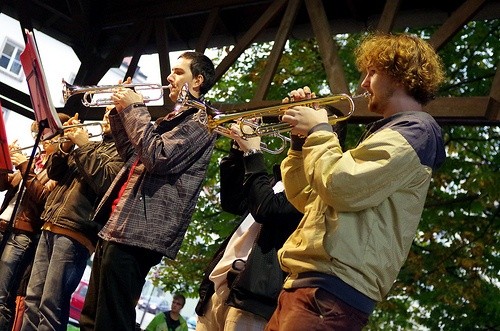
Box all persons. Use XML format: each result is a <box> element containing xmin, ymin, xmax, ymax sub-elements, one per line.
<box><xmin>145</xmin><ymin>294</ymin><xmax>188</xmax><ymax>331</ymax></box>
<box><xmin>0</xmin><ymin>113</ymin><xmax>70</xmax><ymax>331</ymax></box>
<box><xmin>264</xmin><ymin>32</ymin><xmax>446</xmax><ymax>331</ymax></box>
<box><xmin>21</xmin><ymin>104</ymin><xmax>125</xmax><ymax>331</ymax></box>
<box><xmin>196</xmin><ymin>105</ymin><xmax>347</xmax><ymax>331</ymax></box>
<box><xmin>79</xmin><ymin>52</ymin><xmax>216</xmax><ymax>331</ymax></box>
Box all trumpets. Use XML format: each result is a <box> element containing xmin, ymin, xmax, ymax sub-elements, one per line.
<box><xmin>10</xmin><ymin>143</ymin><xmax>45</xmax><ymax>165</ymax></box>
<box><xmin>61</xmin><ymin>79</ymin><xmax>173</xmax><ymax>107</ymax></box>
<box><xmin>205</xmin><ymin>90</ymin><xmax>372</xmax><ymax>139</ymax></box>
<box><xmin>41</xmin><ymin>121</ymin><xmax>109</xmax><ymax>144</ymax></box>
<box><xmin>176</xmin><ymin>82</ymin><xmax>291</xmax><ymax>155</ymax></box>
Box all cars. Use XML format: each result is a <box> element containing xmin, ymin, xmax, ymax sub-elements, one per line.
<box><xmin>67</xmin><ymin>279</ymin><xmax>90</xmax><ymax>324</ymax></box>
<box><xmin>139</xmin><ymin>295</ymin><xmax>169</xmax><ymax>315</ymax></box>
<box><xmin>186</xmin><ymin>317</ymin><xmax>197</xmax><ymax>329</ymax></box>
